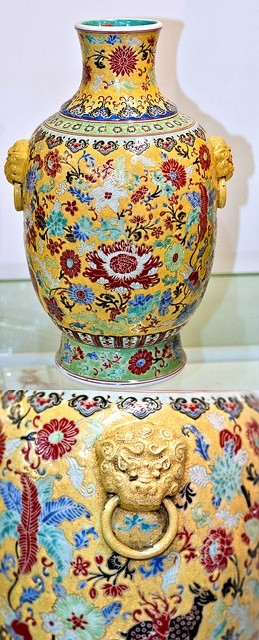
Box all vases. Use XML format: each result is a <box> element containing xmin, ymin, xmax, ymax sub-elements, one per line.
<box><xmin>4</xmin><ymin>19</ymin><xmax>235</xmax><ymax>388</ymax></box>
